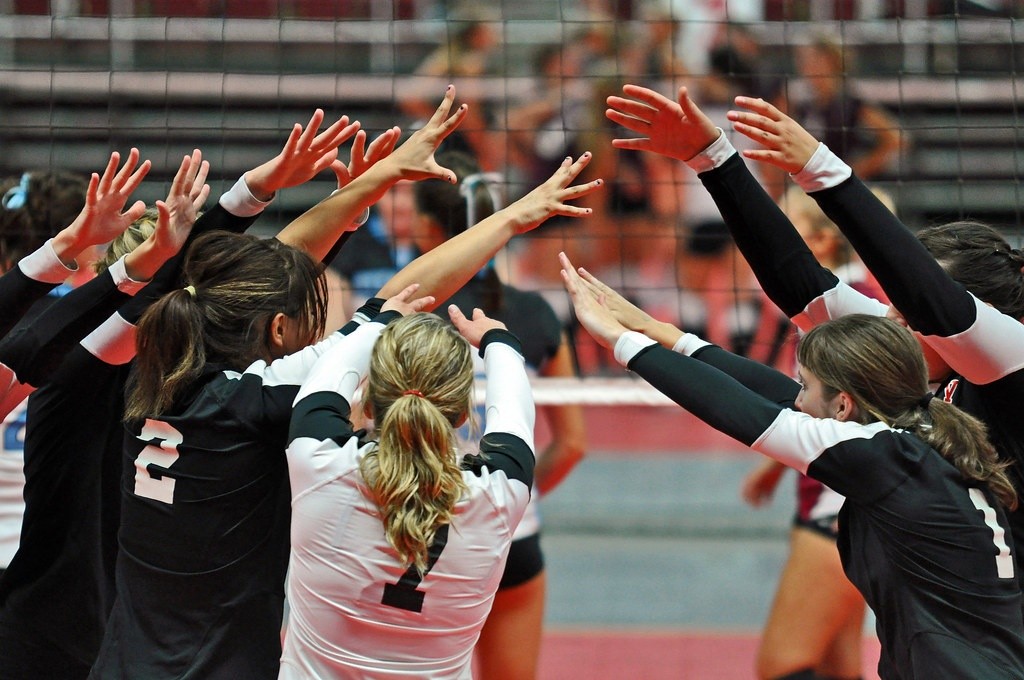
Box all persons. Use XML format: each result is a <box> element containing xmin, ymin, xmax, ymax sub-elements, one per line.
<box><xmin>560</xmin><ymin>252</ymin><xmax>1024</xmax><ymax>680</ymax></box>
<box><xmin>0</xmin><ymin>0</ymin><xmax>1024</xmax><ymax>680</ymax></box>
